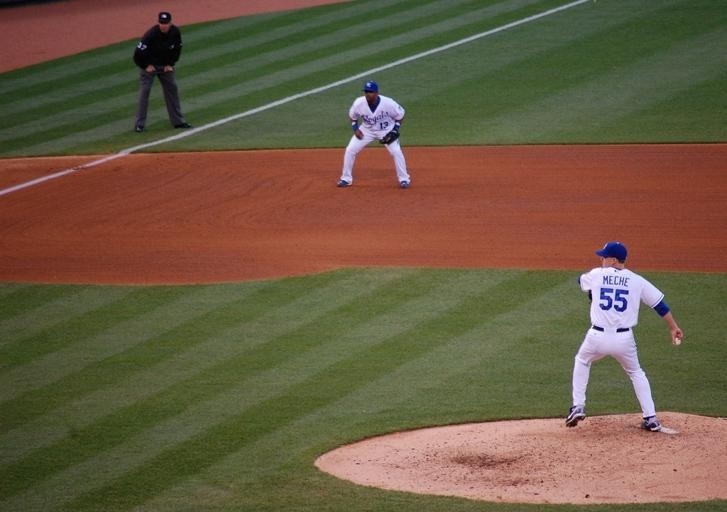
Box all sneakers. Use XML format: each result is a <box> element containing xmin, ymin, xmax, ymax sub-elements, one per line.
<box><xmin>174</xmin><ymin>121</ymin><xmax>192</xmax><ymax>129</ymax></box>
<box><xmin>399</xmin><ymin>180</ymin><xmax>409</xmax><ymax>188</ymax></box>
<box><xmin>565</xmin><ymin>404</ymin><xmax>587</xmax><ymax>429</ymax></box>
<box><xmin>134</xmin><ymin>124</ymin><xmax>144</xmax><ymax>133</ymax></box>
<box><xmin>640</xmin><ymin>417</ymin><xmax>663</xmax><ymax>432</ymax></box>
<box><xmin>336</xmin><ymin>178</ymin><xmax>353</xmax><ymax>187</ymax></box>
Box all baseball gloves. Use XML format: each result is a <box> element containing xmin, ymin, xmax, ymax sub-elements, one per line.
<box><xmin>381</xmin><ymin>129</ymin><xmax>399</xmax><ymax>145</ymax></box>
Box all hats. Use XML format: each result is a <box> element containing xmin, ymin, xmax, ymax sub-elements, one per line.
<box><xmin>360</xmin><ymin>80</ymin><xmax>379</xmax><ymax>93</ymax></box>
<box><xmin>594</xmin><ymin>240</ymin><xmax>628</xmax><ymax>262</ymax></box>
<box><xmin>157</xmin><ymin>11</ymin><xmax>172</xmax><ymax>24</ymax></box>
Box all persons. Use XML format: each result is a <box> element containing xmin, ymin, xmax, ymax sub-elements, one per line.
<box><xmin>337</xmin><ymin>80</ymin><xmax>410</xmax><ymax>190</ymax></box>
<box><xmin>566</xmin><ymin>242</ymin><xmax>683</xmax><ymax>431</ymax></box>
<box><xmin>133</xmin><ymin>12</ymin><xmax>191</xmax><ymax>132</ymax></box>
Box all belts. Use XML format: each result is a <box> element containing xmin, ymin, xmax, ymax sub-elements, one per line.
<box><xmin>591</xmin><ymin>324</ymin><xmax>632</xmax><ymax>333</ymax></box>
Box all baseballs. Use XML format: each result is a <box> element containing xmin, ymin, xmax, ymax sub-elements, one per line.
<box><xmin>672</xmin><ymin>337</ymin><xmax>680</xmax><ymax>345</ymax></box>
